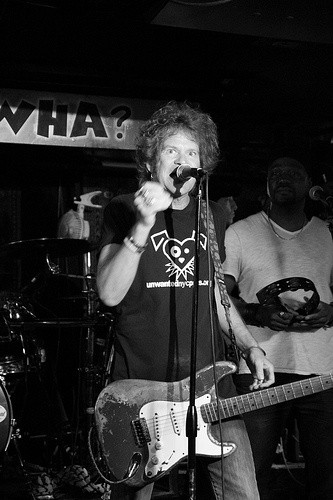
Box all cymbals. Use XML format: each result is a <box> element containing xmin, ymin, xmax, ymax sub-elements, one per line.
<box><xmin>255</xmin><ymin>277</ymin><xmax>320</xmax><ymax>324</ymax></box>
<box><xmin>0</xmin><ymin>238</ymin><xmax>92</xmax><ymax>260</ymax></box>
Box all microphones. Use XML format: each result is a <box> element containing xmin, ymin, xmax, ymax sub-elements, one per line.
<box><xmin>18</xmin><ymin>277</ymin><xmax>37</xmax><ymax>293</ymax></box>
<box><xmin>176</xmin><ymin>163</ymin><xmax>208</xmax><ymax>181</ymax></box>
<box><xmin>309</xmin><ymin>186</ymin><xmax>333</xmax><ymax>209</ymax></box>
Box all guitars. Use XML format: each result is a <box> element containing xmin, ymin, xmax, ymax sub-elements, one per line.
<box><xmin>95</xmin><ymin>361</ymin><xmax>332</xmax><ymax>488</ymax></box>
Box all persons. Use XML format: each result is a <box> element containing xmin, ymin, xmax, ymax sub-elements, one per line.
<box><xmin>222</xmin><ymin>152</ymin><xmax>333</xmax><ymax>500</ymax></box>
<box><xmin>97</xmin><ymin>102</ymin><xmax>275</xmax><ymax>500</ymax></box>
<box><xmin>209</xmin><ymin>183</ymin><xmax>238</xmax><ymax>224</ymax></box>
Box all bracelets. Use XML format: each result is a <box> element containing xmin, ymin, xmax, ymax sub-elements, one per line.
<box><xmin>124</xmin><ymin>231</ymin><xmax>146</xmax><ymax>254</ymax></box>
<box><xmin>241</xmin><ymin>346</ymin><xmax>266</xmax><ymax>361</ymax></box>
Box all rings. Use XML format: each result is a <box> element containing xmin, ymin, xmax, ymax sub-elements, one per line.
<box><xmin>143</xmin><ymin>190</ymin><xmax>149</xmax><ymax>198</ymax></box>
<box><xmin>145</xmin><ymin>197</ymin><xmax>154</xmax><ymax>206</ymax></box>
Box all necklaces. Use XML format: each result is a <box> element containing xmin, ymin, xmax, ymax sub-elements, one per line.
<box><xmin>267</xmin><ymin>207</ymin><xmax>305</xmax><ymax>240</ymax></box>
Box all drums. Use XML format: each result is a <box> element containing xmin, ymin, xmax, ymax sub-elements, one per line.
<box><xmin>0</xmin><ymin>375</ymin><xmax>14</xmax><ymax>468</ymax></box>
<box><xmin>0</xmin><ymin>291</ymin><xmax>46</xmax><ymax>374</ymax></box>
